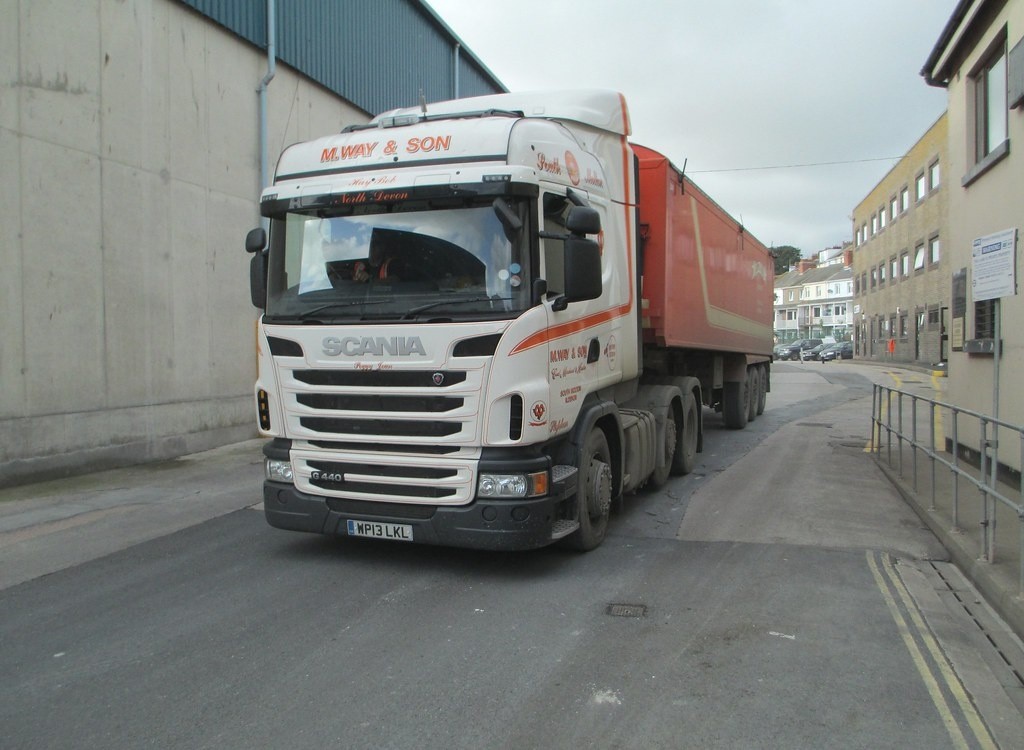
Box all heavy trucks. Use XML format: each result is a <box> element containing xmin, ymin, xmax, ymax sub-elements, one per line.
<box><xmin>246</xmin><ymin>91</ymin><xmax>775</xmax><ymax>554</ymax></box>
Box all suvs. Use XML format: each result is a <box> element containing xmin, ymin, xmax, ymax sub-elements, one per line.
<box><xmin>777</xmin><ymin>339</ymin><xmax>823</xmax><ymax>361</ymax></box>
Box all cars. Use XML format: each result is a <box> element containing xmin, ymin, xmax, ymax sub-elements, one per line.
<box><xmin>773</xmin><ymin>344</ymin><xmax>789</xmax><ymax>360</ymax></box>
<box><xmin>819</xmin><ymin>340</ymin><xmax>853</xmax><ymax>359</ymax></box>
<box><xmin>800</xmin><ymin>343</ymin><xmax>835</xmax><ymax>361</ymax></box>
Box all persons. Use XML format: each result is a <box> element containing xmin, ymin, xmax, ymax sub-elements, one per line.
<box><xmin>345</xmin><ymin>232</ymin><xmax>409</xmax><ymax>283</ymax></box>
